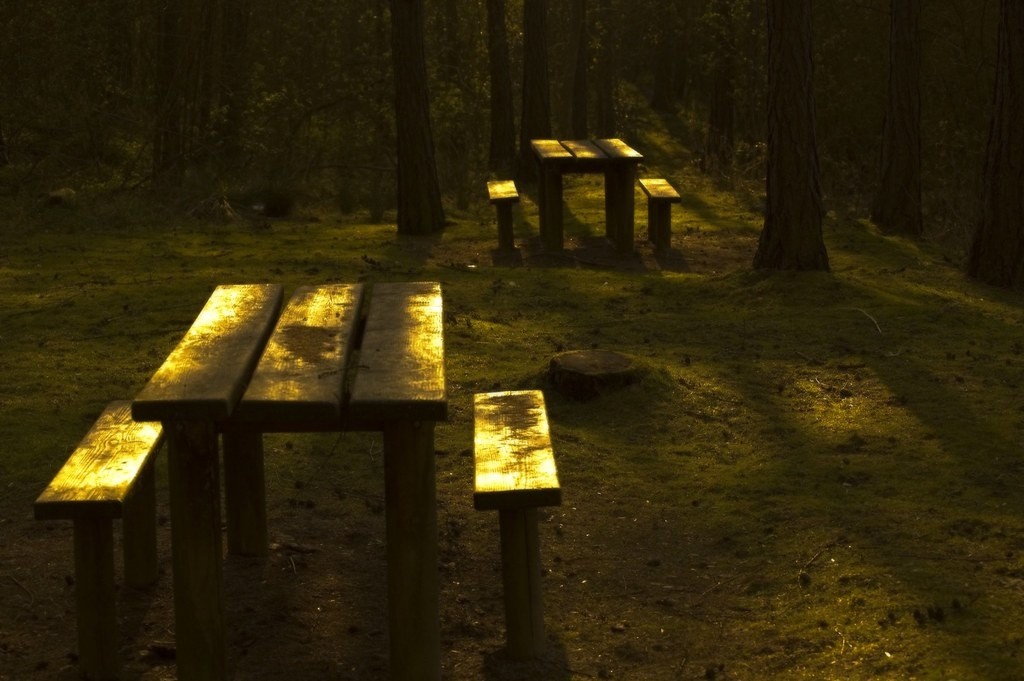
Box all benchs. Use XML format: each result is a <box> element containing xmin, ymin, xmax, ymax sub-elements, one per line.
<box><xmin>473</xmin><ymin>391</ymin><xmax>562</xmax><ymax>660</ymax></box>
<box><xmin>486</xmin><ymin>179</ymin><xmax>520</xmax><ymax>253</ymax></box>
<box><xmin>33</xmin><ymin>401</ymin><xmax>169</xmax><ymax>681</ymax></box>
<box><xmin>639</xmin><ymin>178</ymin><xmax>682</xmax><ymax>250</ymax></box>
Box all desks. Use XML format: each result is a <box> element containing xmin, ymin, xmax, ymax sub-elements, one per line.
<box><xmin>530</xmin><ymin>137</ymin><xmax>644</xmax><ymax>253</ymax></box>
<box><xmin>130</xmin><ymin>281</ymin><xmax>447</xmax><ymax>681</ymax></box>
